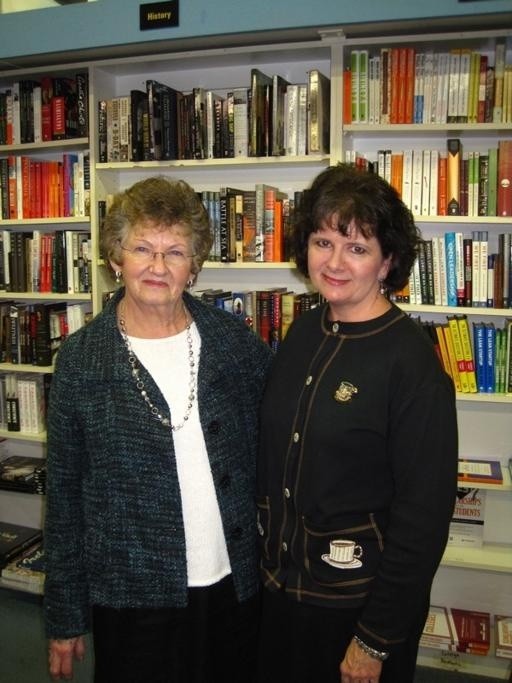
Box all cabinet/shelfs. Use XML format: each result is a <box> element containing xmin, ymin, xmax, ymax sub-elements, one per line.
<box><xmin>2</xmin><ymin>26</ymin><xmax>512</xmax><ymax>680</ymax></box>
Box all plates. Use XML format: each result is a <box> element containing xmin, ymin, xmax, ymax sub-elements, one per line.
<box><xmin>320</xmin><ymin>553</ymin><xmax>362</xmax><ymax>570</ymax></box>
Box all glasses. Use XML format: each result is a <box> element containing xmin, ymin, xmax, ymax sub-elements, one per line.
<box><xmin>120</xmin><ymin>244</ymin><xmax>197</xmax><ymax>261</ymax></box>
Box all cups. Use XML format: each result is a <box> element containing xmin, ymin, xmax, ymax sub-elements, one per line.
<box><xmin>328</xmin><ymin>539</ymin><xmax>365</xmax><ymax>564</ymax></box>
